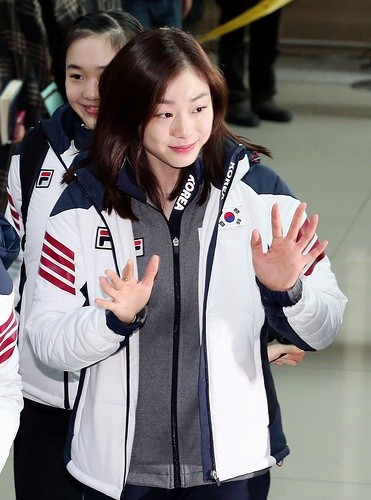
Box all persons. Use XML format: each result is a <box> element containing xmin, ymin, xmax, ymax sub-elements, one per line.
<box><xmin>0</xmin><ymin>187</ymin><xmax>24</xmax><ymax>500</ymax></box>
<box><xmin>0</xmin><ymin>0</ymin><xmax>293</xmax><ymax>215</ymax></box>
<box><xmin>0</xmin><ymin>9</ymin><xmax>305</xmax><ymax>500</ymax></box>
<box><xmin>24</xmin><ymin>26</ymin><xmax>348</xmax><ymax>500</ymax></box>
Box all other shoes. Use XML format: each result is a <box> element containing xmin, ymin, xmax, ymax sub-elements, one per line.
<box><xmin>224</xmin><ymin>102</ymin><xmax>291</xmax><ymax>127</ymax></box>
